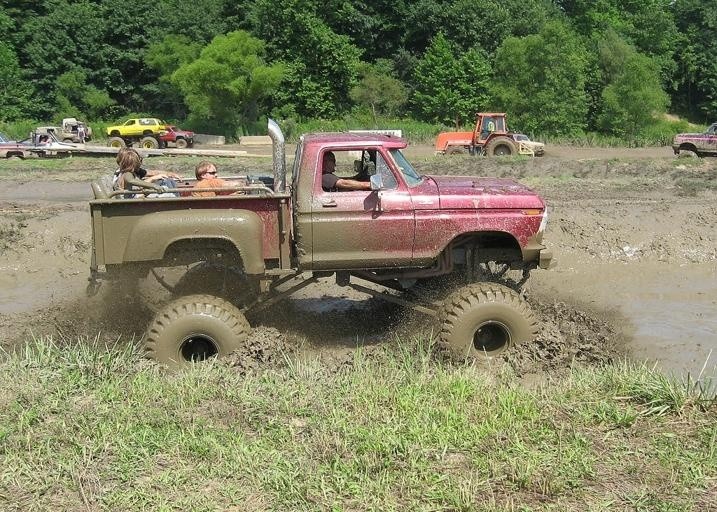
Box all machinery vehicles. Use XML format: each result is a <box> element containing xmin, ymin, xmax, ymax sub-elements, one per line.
<box><xmin>431</xmin><ymin>111</ymin><xmax>521</xmax><ymax>159</ymax></box>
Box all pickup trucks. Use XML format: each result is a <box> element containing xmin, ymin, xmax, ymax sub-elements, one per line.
<box><xmin>0</xmin><ymin>116</ymin><xmax>93</xmax><ymax>159</ymax></box>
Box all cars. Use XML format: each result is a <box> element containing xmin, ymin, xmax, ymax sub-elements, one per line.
<box><xmin>508</xmin><ymin>131</ymin><xmax>545</xmax><ymax>157</ymax></box>
<box><xmin>667</xmin><ymin>121</ymin><xmax>717</xmax><ymax>158</ymax></box>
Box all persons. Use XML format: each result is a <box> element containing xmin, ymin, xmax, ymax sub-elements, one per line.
<box><xmin>112</xmin><ymin>147</ymin><xmax>183</xmax><ymax>199</ymax></box>
<box><xmin>77</xmin><ymin>124</ymin><xmax>85</xmax><ymax>143</ymax></box>
<box><xmin>40</xmin><ymin>137</ymin><xmax>48</xmax><ymax>144</ymax></box>
<box><xmin>159</xmin><ymin>120</ymin><xmax>166</xmax><ymax>148</ymax></box>
<box><xmin>190</xmin><ymin>161</ymin><xmax>245</xmax><ymax>196</ymax></box>
<box><xmin>323</xmin><ymin>152</ymin><xmax>370</xmax><ymax>192</ymax></box>
<box><xmin>480</xmin><ymin>118</ymin><xmax>495</xmax><ymax>140</ymax></box>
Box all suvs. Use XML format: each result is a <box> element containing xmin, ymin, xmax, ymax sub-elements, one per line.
<box><xmin>159</xmin><ymin>122</ymin><xmax>197</xmax><ymax>148</ymax></box>
<box><xmin>105</xmin><ymin>116</ymin><xmax>167</xmax><ymax>148</ymax></box>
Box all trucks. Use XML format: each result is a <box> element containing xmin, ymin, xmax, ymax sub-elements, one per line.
<box><xmin>85</xmin><ymin>115</ymin><xmax>552</xmax><ymax>376</ymax></box>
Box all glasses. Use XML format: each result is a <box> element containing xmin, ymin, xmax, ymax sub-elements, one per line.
<box><xmin>203</xmin><ymin>170</ymin><xmax>218</xmax><ymax>176</ymax></box>
